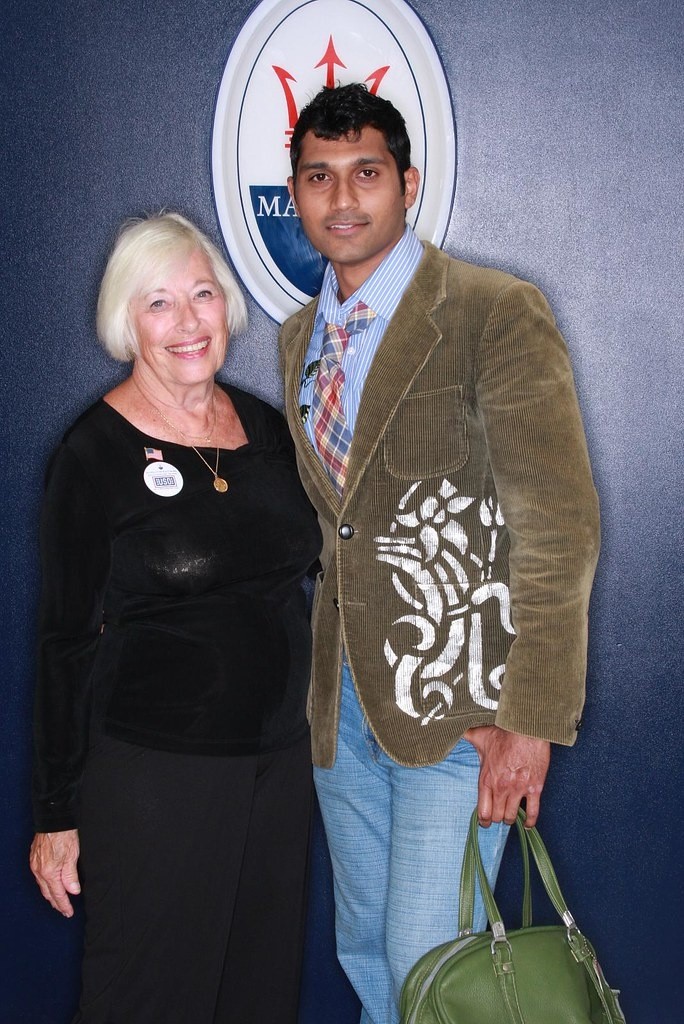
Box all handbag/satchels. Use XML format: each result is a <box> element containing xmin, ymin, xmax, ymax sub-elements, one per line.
<box><xmin>399</xmin><ymin>805</ymin><xmax>626</xmax><ymax>1024</ymax></box>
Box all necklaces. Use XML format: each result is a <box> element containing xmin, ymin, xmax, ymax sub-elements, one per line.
<box><xmin>131</xmin><ymin>376</ymin><xmax>228</xmax><ymax>494</ymax></box>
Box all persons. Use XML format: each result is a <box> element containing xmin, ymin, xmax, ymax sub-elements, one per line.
<box><xmin>27</xmin><ymin>195</ymin><xmax>325</xmax><ymax>1024</ymax></box>
<box><xmin>277</xmin><ymin>81</ymin><xmax>603</xmax><ymax>1024</ymax></box>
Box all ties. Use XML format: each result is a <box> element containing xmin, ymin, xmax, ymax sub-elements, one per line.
<box><xmin>310</xmin><ymin>300</ymin><xmax>378</xmax><ymax>503</ymax></box>
